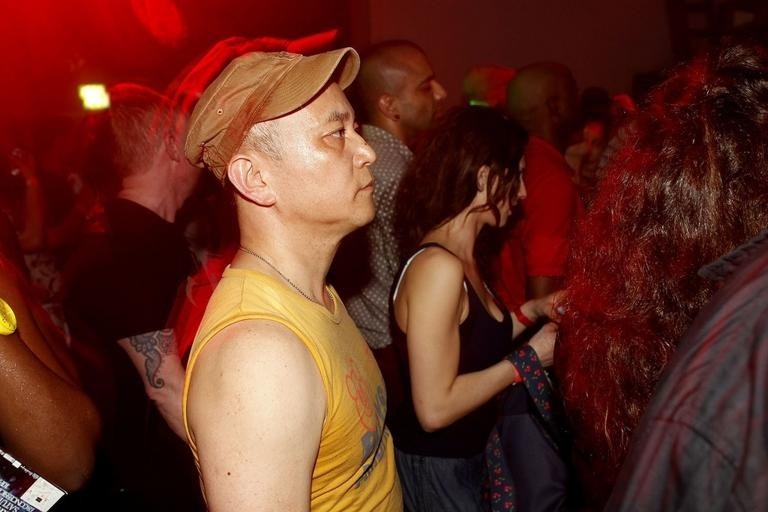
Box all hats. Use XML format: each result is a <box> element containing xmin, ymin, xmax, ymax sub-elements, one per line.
<box><xmin>183</xmin><ymin>45</ymin><xmax>362</xmax><ymax>190</ymax></box>
<box><xmin>174</xmin><ymin>28</ymin><xmax>344</xmax><ymax>169</ymax></box>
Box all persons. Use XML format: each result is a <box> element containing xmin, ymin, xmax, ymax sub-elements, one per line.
<box><xmin>2</xmin><ymin>2</ymin><xmax>765</xmax><ymax>511</ymax></box>
